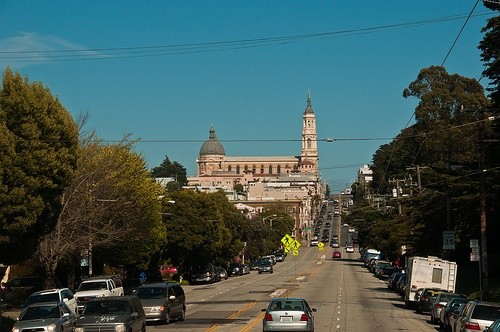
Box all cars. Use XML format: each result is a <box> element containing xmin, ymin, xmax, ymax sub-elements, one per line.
<box><xmin>71</xmin><ymin>295</ymin><xmax>146</xmax><ymax>332</ymax></box>
<box><xmin>130</xmin><ymin>282</ymin><xmax>187</xmax><ymax>325</ymax></box>
<box><xmin>261</xmin><ymin>297</ymin><xmax>317</xmax><ymax>332</ymax></box>
<box><xmin>20</xmin><ymin>287</ymin><xmax>79</xmax><ymax>316</ymax></box>
<box><xmin>333</xmin><ymin>250</ymin><xmax>341</xmax><ymax>258</ymax></box>
<box><xmin>310</xmin><ymin>200</ymin><xmax>355</xmax><ymax>253</ymax></box>
<box><xmin>363</xmin><ymin>248</ymin><xmax>500</xmax><ymax>332</ymax></box>
<box><xmin>190</xmin><ymin>247</ymin><xmax>288</xmax><ymax>285</ymax></box>
<box><xmin>11</xmin><ymin>301</ymin><xmax>78</xmax><ymax>332</ymax></box>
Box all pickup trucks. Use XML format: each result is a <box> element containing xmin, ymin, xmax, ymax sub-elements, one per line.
<box><xmin>73</xmin><ymin>278</ymin><xmax>125</xmax><ymax>314</ymax></box>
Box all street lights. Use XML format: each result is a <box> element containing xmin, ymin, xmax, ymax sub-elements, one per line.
<box><xmin>262</xmin><ymin>214</ymin><xmax>276</xmax><ymax>242</ymax></box>
<box><xmin>269</xmin><ymin>216</ymin><xmax>283</xmax><ymax>230</ymax></box>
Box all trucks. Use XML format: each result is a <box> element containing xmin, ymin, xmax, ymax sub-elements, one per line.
<box><xmin>403</xmin><ymin>256</ymin><xmax>458</xmax><ymax>309</ymax></box>
